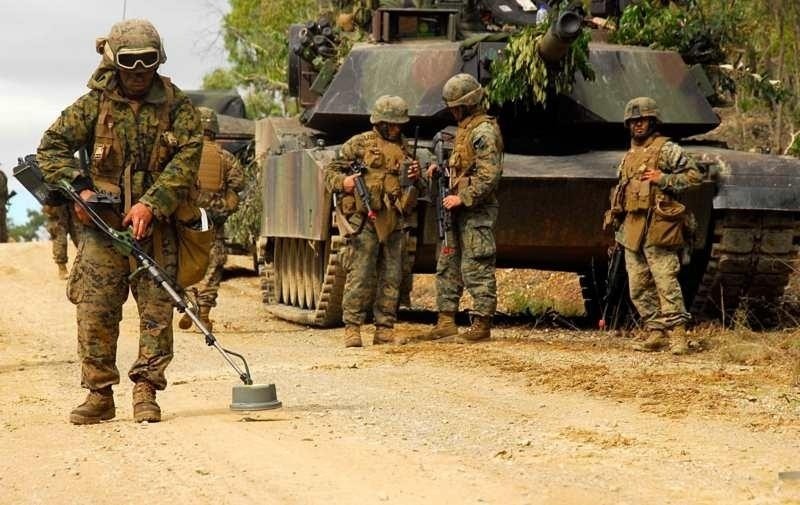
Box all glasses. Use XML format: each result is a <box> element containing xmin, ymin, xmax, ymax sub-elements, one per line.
<box><xmin>103</xmin><ymin>40</ymin><xmax>160</xmax><ymax>69</ymax></box>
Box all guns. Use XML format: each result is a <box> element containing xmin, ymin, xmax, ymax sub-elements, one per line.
<box><xmin>427</xmin><ymin>131</ymin><xmax>454</xmax><ymax>258</ymax></box>
<box><xmin>5</xmin><ymin>189</ymin><xmax>19</xmax><ymax>212</ymax></box>
<box><xmin>347</xmin><ymin>162</ymin><xmax>377</xmax><ymax>225</ymax></box>
<box><xmin>597</xmin><ymin>238</ymin><xmax>623</xmax><ymax>329</ymax></box>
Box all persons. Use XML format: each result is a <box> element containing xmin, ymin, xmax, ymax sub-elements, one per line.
<box><xmin>179</xmin><ymin>107</ymin><xmax>243</xmax><ymax>334</ymax></box>
<box><xmin>604</xmin><ymin>97</ymin><xmax>701</xmax><ymax>354</ymax></box>
<box><xmin>425</xmin><ymin>73</ymin><xmax>503</xmax><ymax>343</ymax></box>
<box><xmin>43</xmin><ymin>156</ymin><xmax>84</xmax><ymax>276</ymax></box>
<box><xmin>0</xmin><ymin>171</ymin><xmax>15</xmax><ymax>242</ymax></box>
<box><xmin>34</xmin><ymin>17</ymin><xmax>202</xmax><ymax>423</ymax></box>
<box><xmin>324</xmin><ymin>94</ymin><xmax>422</xmax><ymax>348</ymax></box>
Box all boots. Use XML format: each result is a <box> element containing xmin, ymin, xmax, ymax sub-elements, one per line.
<box><xmin>456</xmin><ymin>314</ymin><xmax>491</xmax><ymax>343</ymax></box>
<box><xmin>631</xmin><ymin>328</ymin><xmax>666</xmax><ymax>351</ymax></box>
<box><xmin>70</xmin><ymin>385</ymin><xmax>115</xmax><ymax>425</ymax></box>
<box><xmin>132</xmin><ymin>379</ymin><xmax>161</xmax><ymax>423</ymax></box>
<box><xmin>426</xmin><ymin>312</ymin><xmax>458</xmax><ymax>340</ymax></box>
<box><xmin>344</xmin><ymin>323</ymin><xmax>362</xmax><ymax>347</ymax></box>
<box><xmin>373</xmin><ymin>324</ymin><xmax>394</xmax><ymax>345</ymax></box>
<box><xmin>58</xmin><ymin>262</ymin><xmax>213</xmax><ymax>333</ymax></box>
<box><xmin>669</xmin><ymin>323</ymin><xmax>686</xmax><ymax>355</ymax></box>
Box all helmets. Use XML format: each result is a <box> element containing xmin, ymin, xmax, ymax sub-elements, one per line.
<box><xmin>104</xmin><ymin>18</ymin><xmax>167</xmax><ymax>72</ymax></box>
<box><xmin>623</xmin><ymin>96</ymin><xmax>664</xmax><ymax>127</ymax></box>
<box><xmin>370</xmin><ymin>94</ymin><xmax>410</xmax><ymax>124</ymax></box>
<box><xmin>442</xmin><ymin>72</ymin><xmax>482</xmax><ymax>107</ymax></box>
<box><xmin>195</xmin><ymin>104</ymin><xmax>219</xmax><ymax>134</ymax></box>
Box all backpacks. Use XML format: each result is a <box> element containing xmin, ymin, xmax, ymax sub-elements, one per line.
<box><xmin>680</xmin><ymin>151</ymin><xmax>720</xmax><ymax>248</ymax></box>
